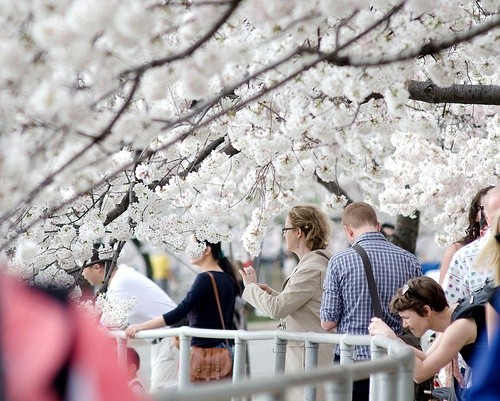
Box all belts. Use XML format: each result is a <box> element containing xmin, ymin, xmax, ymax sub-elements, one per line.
<box><xmin>153</xmin><ymin>338</ymin><xmax>163</xmax><ymax>345</ymax></box>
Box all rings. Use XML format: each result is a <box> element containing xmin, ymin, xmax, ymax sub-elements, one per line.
<box><xmin>246</xmin><ymin>273</ymin><xmax>250</xmax><ymax>276</ymax></box>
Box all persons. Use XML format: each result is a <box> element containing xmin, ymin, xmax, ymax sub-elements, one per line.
<box><xmin>439</xmin><ymin>186</ymin><xmax>495</xmax><ymax>286</ymax></box>
<box><xmin>125</xmin><ymin>347</ymin><xmax>147</xmax><ymax>400</ymax></box>
<box><xmin>436</xmin><ymin>186</ymin><xmax>500</xmax><ymax>401</ymax></box>
<box><xmin>368</xmin><ymin>276</ymin><xmax>486</xmax><ymax>384</ymax></box>
<box><xmin>82</xmin><ymin>243</ymin><xmax>178</xmax><ymax>401</ymax></box>
<box><xmin>463</xmin><ymin>211</ymin><xmax>500</xmax><ymax>401</ymax></box>
<box><xmin>320</xmin><ymin>202</ymin><xmax>424</xmax><ymax>401</ymax></box>
<box><xmin>126</xmin><ymin>230</ymin><xmax>244</xmax><ymax>384</ymax></box>
<box><xmin>238</xmin><ymin>205</ymin><xmax>338</xmax><ymax>401</ymax></box>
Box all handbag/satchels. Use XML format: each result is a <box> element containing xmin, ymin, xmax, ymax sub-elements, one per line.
<box><xmin>189</xmin><ymin>345</ymin><xmax>232</xmax><ymax>382</ymax></box>
<box><xmin>399</xmin><ymin>334</ymin><xmax>431</xmax><ymax>400</ymax></box>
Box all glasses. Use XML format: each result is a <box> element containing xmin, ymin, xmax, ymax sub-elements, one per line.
<box><xmin>402</xmin><ymin>283</ymin><xmax>432</xmax><ymax>309</ymax></box>
<box><xmin>282</xmin><ymin>228</ymin><xmax>295</xmax><ymax>234</ymax></box>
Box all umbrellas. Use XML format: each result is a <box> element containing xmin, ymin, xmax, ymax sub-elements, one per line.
<box><xmin>424</xmin><ymin>387</ymin><xmax>450</xmax><ymax>399</ymax></box>
